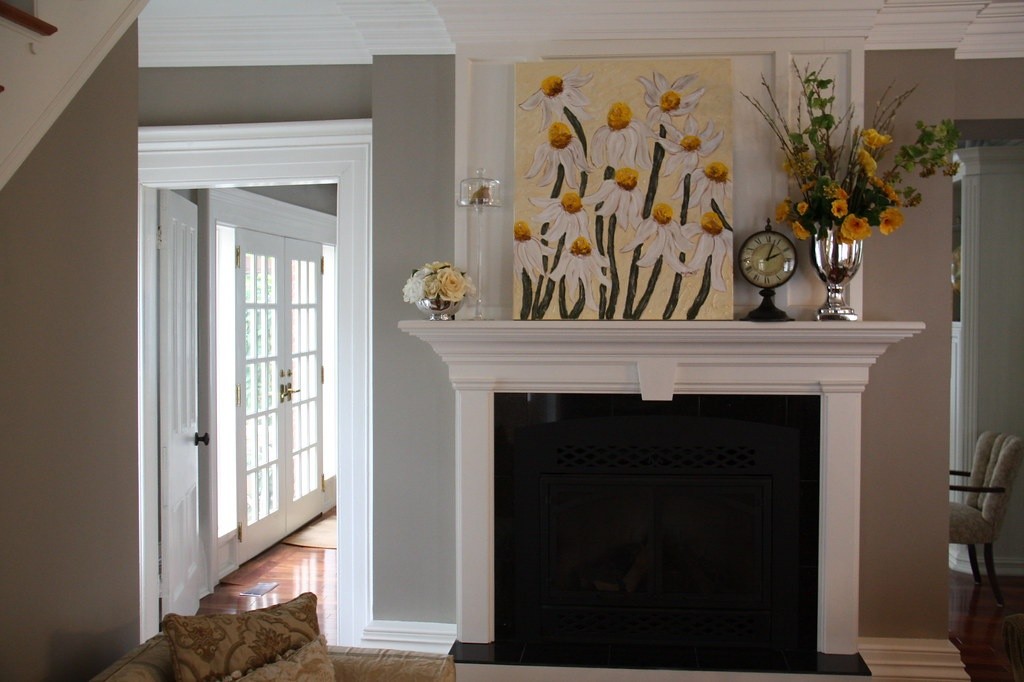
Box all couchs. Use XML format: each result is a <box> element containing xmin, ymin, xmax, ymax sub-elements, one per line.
<box><xmin>92</xmin><ymin>630</ymin><xmax>455</xmax><ymax>682</ymax></box>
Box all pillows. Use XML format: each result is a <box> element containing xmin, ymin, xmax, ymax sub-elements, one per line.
<box><xmin>236</xmin><ymin>636</ymin><xmax>335</xmax><ymax>682</ymax></box>
<box><xmin>163</xmin><ymin>593</ymin><xmax>318</xmax><ymax>682</ymax></box>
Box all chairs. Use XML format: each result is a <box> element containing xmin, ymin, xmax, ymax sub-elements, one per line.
<box><xmin>948</xmin><ymin>432</ymin><xmax>1023</xmax><ymax>604</ymax></box>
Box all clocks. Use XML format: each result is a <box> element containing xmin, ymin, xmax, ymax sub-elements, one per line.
<box><xmin>739</xmin><ymin>218</ymin><xmax>797</xmax><ymax>322</ymax></box>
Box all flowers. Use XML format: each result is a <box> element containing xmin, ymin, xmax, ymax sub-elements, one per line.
<box><xmin>754</xmin><ymin>87</ymin><xmax>965</xmax><ymax>244</ymax></box>
<box><xmin>402</xmin><ymin>261</ymin><xmax>475</xmax><ymax>304</ymax></box>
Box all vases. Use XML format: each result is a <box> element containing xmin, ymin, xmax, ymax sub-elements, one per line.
<box><xmin>412</xmin><ymin>297</ymin><xmax>463</xmax><ymax>321</ymax></box>
<box><xmin>809</xmin><ymin>219</ymin><xmax>864</xmax><ymax>322</ymax></box>
